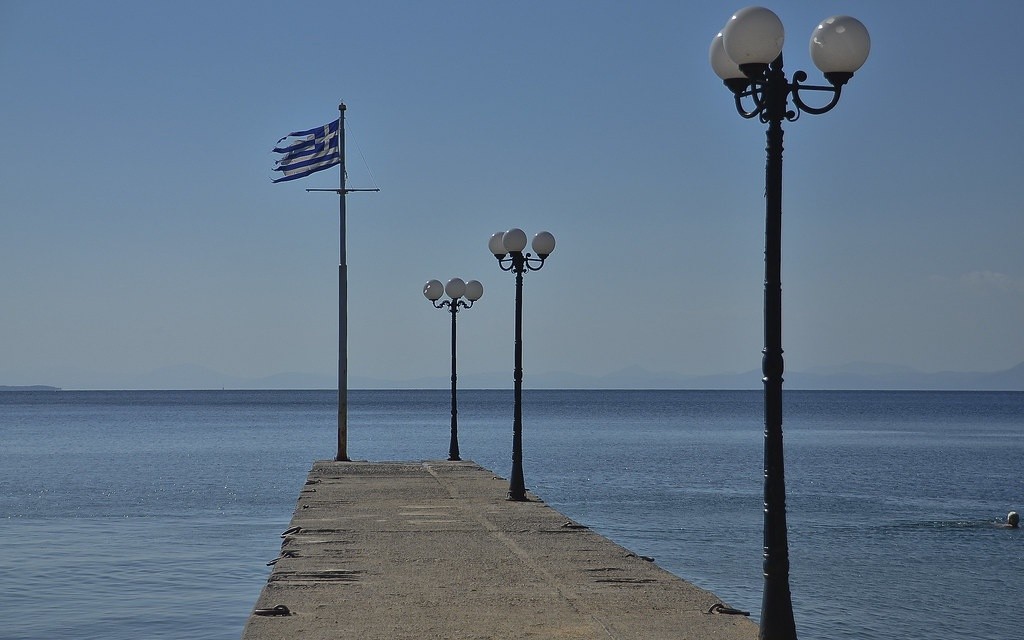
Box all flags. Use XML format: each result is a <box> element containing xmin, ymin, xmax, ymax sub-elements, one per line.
<box><xmin>268</xmin><ymin>119</ymin><xmax>342</xmax><ymax>183</ymax></box>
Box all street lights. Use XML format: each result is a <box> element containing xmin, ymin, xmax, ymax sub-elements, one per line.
<box><xmin>488</xmin><ymin>229</ymin><xmax>556</xmax><ymax>501</ymax></box>
<box><xmin>423</xmin><ymin>278</ymin><xmax>483</xmax><ymax>460</ymax></box>
<box><xmin>707</xmin><ymin>5</ymin><xmax>871</xmax><ymax>640</ymax></box>
